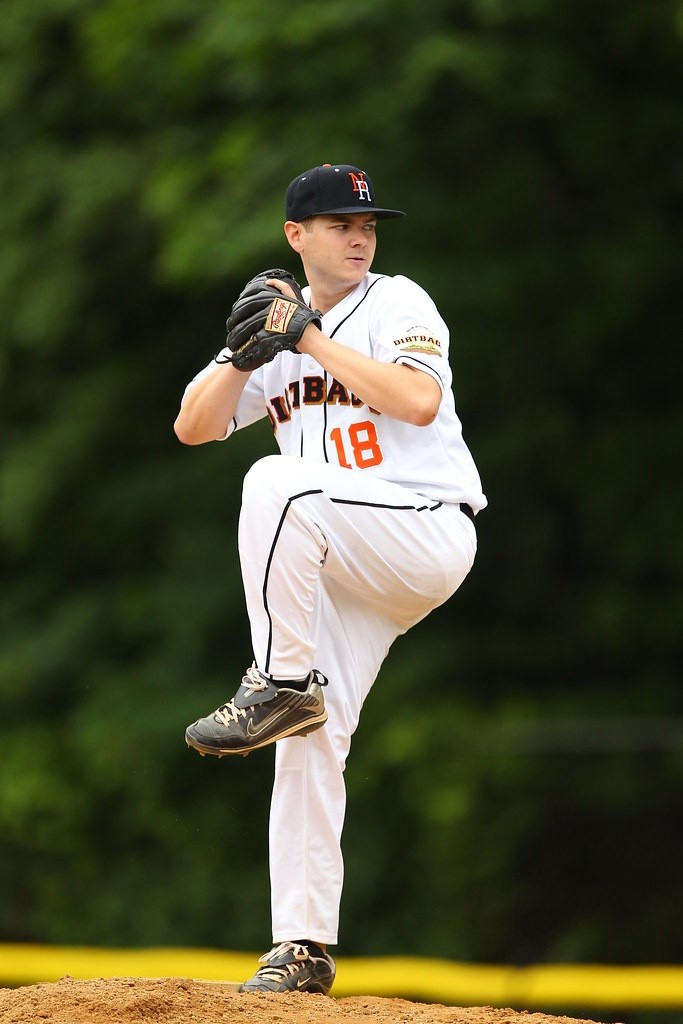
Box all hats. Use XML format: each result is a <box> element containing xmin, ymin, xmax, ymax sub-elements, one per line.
<box><xmin>285</xmin><ymin>161</ymin><xmax>406</xmax><ymax>223</ymax></box>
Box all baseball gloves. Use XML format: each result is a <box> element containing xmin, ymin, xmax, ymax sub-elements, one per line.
<box><xmin>227</xmin><ymin>267</ymin><xmax>324</xmax><ymax>371</ymax></box>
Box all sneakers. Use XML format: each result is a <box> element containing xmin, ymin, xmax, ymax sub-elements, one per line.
<box><xmin>184</xmin><ymin>659</ymin><xmax>330</xmax><ymax>756</ymax></box>
<box><xmin>240</xmin><ymin>940</ymin><xmax>336</xmax><ymax>995</ymax></box>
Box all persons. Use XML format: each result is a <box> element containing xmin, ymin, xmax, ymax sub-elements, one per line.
<box><xmin>173</xmin><ymin>165</ymin><xmax>489</xmax><ymax>993</ymax></box>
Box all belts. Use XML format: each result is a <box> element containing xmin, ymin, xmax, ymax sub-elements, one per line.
<box><xmin>461</xmin><ymin>501</ymin><xmax>477</xmax><ymax>526</ymax></box>
